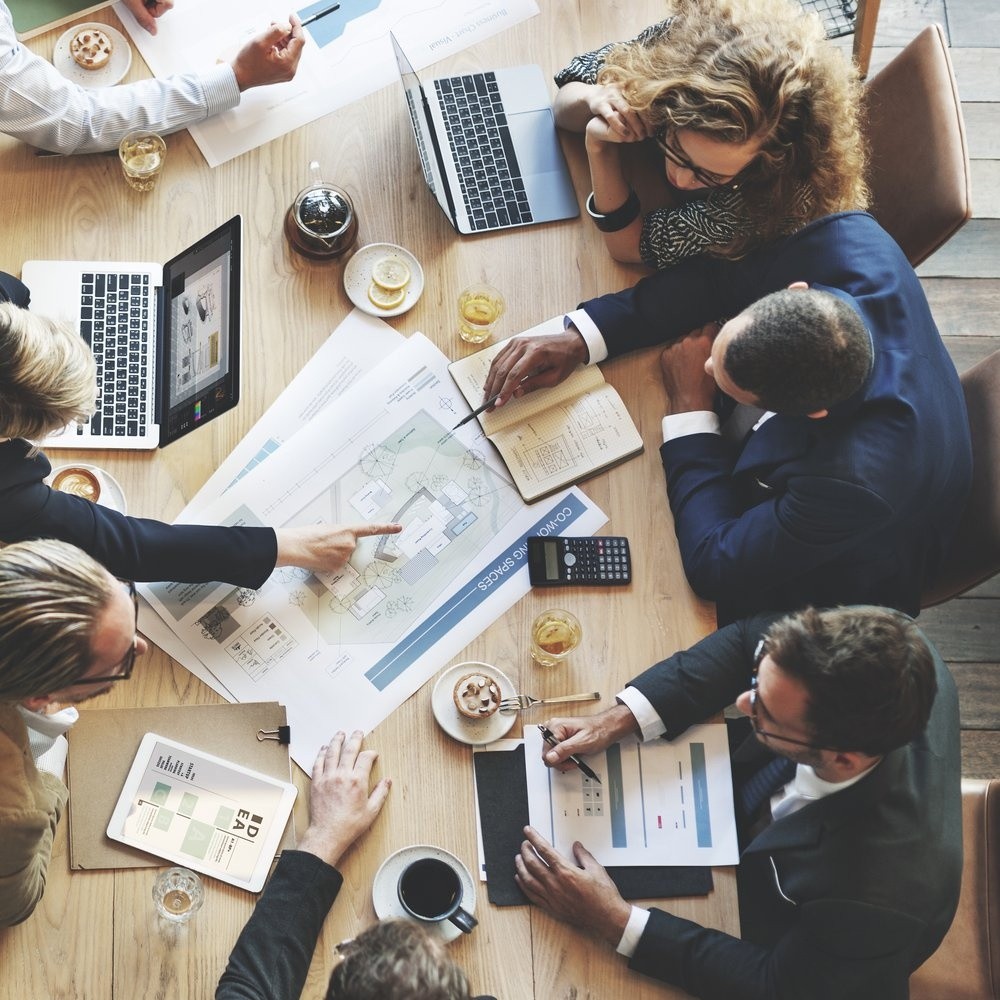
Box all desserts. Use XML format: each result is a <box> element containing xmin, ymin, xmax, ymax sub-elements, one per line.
<box><xmin>69</xmin><ymin>28</ymin><xmax>112</xmax><ymax>70</ymax></box>
<box><xmin>454</xmin><ymin>673</ymin><xmax>502</xmax><ymax>719</ymax></box>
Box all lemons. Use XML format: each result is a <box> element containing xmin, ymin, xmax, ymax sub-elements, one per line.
<box><xmin>461</xmin><ymin>300</ymin><xmax>495</xmax><ymax>325</ymax></box>
<box><xmin>369</xmin><ymin>257</ymin><xmax>411</xmax><ymax>309</ymax></box>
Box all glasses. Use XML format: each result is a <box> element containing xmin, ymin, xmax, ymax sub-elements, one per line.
<box><xmin>72</xmin><ymin>576</ymin><xmax>139</xmax><ymax>685</ymax></box>
<box><xmin>751</xmin><ymin>639</ymin><xmax>836</xmax><ymax>756</ymax></box>
<box><xmin>655</xmin><ymin>128</ymin><xmax>722</xmax><ymax>189</ymax></box>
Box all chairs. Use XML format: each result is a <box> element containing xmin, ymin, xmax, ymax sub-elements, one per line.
<box><xmin>856</xmin><ymin>20</ymin><xmax>1000</xmax><ymax>1000</ymax></box>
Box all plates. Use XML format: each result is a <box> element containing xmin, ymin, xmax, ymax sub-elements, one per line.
<box><xmin>343</xmin><ymin>242</ymin><xmax>425</xmax><ymax>318</ymax></box>
<box><xmin>43</xmin><ymin>463</ymin><xmax>128</xmax><ymax>516</ymax></box>
<box><xmin>52</xmin><ymin>21</ymin><xmax>132</xmax><ymax>90</ymax></box>
<box><xmin>372</xmin><ymin>844</ymin><xmax>477</xmax><ymax>946</ymax></box>
<box><xmin>431</xmin><ymin>661</ymin><xmax>518</xmax><ymax>745</ymax></box>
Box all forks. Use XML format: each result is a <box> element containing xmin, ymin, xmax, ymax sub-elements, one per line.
<box><xmin>498</xmin><ymin>692</ymin><xmax>601</xmax><ymax>711</ymax></box>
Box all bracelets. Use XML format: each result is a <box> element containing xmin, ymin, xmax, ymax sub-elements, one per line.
<box><xmin>586</xmin><ymin>186</ymin><xmax>641</xmax><ymax>233</ymax></box>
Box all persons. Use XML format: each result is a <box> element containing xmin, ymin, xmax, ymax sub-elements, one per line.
<box><xmin>482</xmin><ymin>205</ymin><xmax>975</xmax><ymax>626</ymax></box>
<box><xmin>554</xmin><ymin>0</ymin><xmax>873</xmax><ymax>271</ymax></box>
<box><xmin>1</xmin><ymin>540</ymin><xmax>149</xmax><ymax>929</ymax></box>
<box><xmin>213</xmin><ymin>730</ymin><xmax>498</xmax><ymax>1000</ymax></box>
<box><xmin>515</xmin><ymin>603</ymin><xmax>963</xmax><ymax>999</ymax></box>
<box><xmin>0</xmin><ymin>0</ymin><xmax>305</xmax><ymax>158</ymax></box>
<box><xmin>0</xmin><ymin>271</ymin><xmax>403</xmax><ymax>592</ymax></box>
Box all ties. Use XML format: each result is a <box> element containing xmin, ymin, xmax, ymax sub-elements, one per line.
<box><xmin>719</xmin><ymin>403</ymin><xmax>768</xmax><ymax>460</ymax></box>
<box><xmin>738</xmin><ymin>755</ymin><xmax>798</xmax><ymax>834</ymax></box>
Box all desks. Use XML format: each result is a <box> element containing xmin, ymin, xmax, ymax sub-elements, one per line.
<box><xmin>0</xmin><ymin>0</ymin><xmax>744</xmax><ymax>1000</ymax></box>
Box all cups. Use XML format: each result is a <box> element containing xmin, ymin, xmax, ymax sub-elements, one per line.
<box><xmin>530</xmin><ymin>608</ymin><xmax>583</xmax><ymax>667</ymax></box>
<box><xmin>50</xmin><ymin>467</ymin><xmax>101</xmax><ymax>505</ymax></box>
<box><xmin>397</xmin><ymin>858</ymin><xmax>479</xmax><ymax>934</ymax></box>
<box><xmin>457</xmin><ymin>282</ymin><xmax>505</xmax><ymax>344</ymax></box>
<box><xmin>118</xmin><ymin>129</ymin><xmax>167</xmax><ymax>193</ymax></box>
<box><xmin>152</xmin><ymin>867</ymin><xmax>205</xmax><ymax>923</ymax></box>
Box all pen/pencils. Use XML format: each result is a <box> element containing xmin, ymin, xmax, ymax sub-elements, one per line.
<box><xmin>538</xmin><ymin>724</ymin><xmax>601</xmax><ymax>784</ymax></box>
<box><xmin>301</xmin><ymin>2</ymin><xmax>340</xmax><ymax>27</ymax></box>
<box><xmin>452</xmin><ymin>375</ymin><xmax>528</xmax><ymax>431</ymax></box>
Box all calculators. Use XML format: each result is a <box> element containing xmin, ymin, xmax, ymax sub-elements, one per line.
<box><xmin>527</xmin><ymin>536</ymin><xmax>631</xmax><ymax>586</ymax></box>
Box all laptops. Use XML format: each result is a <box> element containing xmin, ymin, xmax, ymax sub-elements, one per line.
<box><xmin>19</xmin><ymin>214</ymin><xmax>241</xmax><ymax>451</ymax></box>
<box><xmin>389</xmin><ymin>30</ymin><xmax>581</xmax><ymax>236</ymax></box>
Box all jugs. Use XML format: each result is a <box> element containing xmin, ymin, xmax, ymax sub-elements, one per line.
<box><xmin>282</xmin><ymin>160</ymin><xmax>359</xmax><ymax>261</ymax></box>
